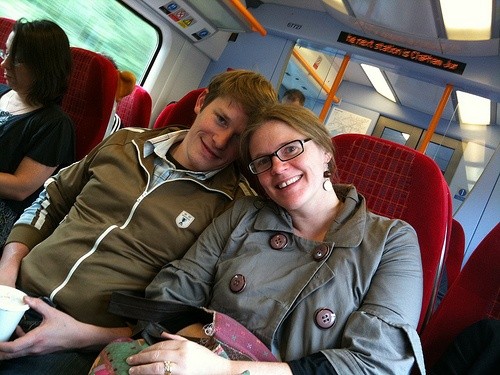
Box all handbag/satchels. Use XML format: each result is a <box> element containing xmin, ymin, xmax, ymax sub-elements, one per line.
<box><xmin>88</xmin><ymin>292</ymin><xmax>280</xmax><ymax>375</ymax></box>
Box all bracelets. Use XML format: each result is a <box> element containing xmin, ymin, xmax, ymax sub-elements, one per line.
<box><xmin>164</xmin><ymin>361</ymin><xmax>171</xmax><ymax>375</ymax></box>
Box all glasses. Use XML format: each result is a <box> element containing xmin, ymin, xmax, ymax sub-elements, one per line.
<box><xmin>0</xmin><ymin>49</ymin><xmax>27</xmax><ymax>65</ymax></box>
<box><xmin>248</xmin><ymin>138</ymin><xmax>313</xmax><ymax>175</ymax></box>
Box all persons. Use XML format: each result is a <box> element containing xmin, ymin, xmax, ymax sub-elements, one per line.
<box><xmin>281</xmin><ymin>88</ymin><xmax>305</xmax><ymax>106</ymax></box>
<box><xmin>0</xmin><ymin>65</ymin><xmax>282</xmax><ymax>375</ymax></box>
<box><xmin>0</xmin><ymin>17</ymin><xmax>76</xmax><ymax>252</ymax></box>
<box><xmin>125</xmin><ymin>104</ymin><xmax>426</xmax><ymax>375</ymax></box>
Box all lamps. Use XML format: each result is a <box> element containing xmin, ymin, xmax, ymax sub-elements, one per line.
<box><xmin>359</xmin><ymin>63</ymin><xmax>401</xmax><ymax>104</ymax></box>
<box><xmin>451</xmin><ymin>89</ymin><xmax>497</xmax><ymax>126</ymax></box>
<box><xmin>322</xmin><ymin>0</ymin><xmax>356</xmax><ymax>18</ymax></box>
<box><xmin>430</xmin><ymin>0</ymin><xmax>500</xmax><ymax>41</ymax></box>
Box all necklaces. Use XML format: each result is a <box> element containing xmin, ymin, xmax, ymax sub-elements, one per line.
<box><xmin>5</xmin><ymin>92</ymin><xmax>34</xmax><ymax>112</ymax></box>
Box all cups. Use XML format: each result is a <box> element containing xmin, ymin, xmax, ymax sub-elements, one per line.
<box><xmin>0</xmin><ymin>284</ymin><xmax>31</xmax><ymax>342</ymax></box>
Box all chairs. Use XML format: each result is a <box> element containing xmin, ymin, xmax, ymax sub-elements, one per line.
<box><xmin>328</xmin><ymin>133</ymin><xmax>500</xmax><ymax>375</ymax></box>
<box><xmin>0</xmin><ymin>17</ymin><xmax>208</xmax><ymax>159</ymax></box>
